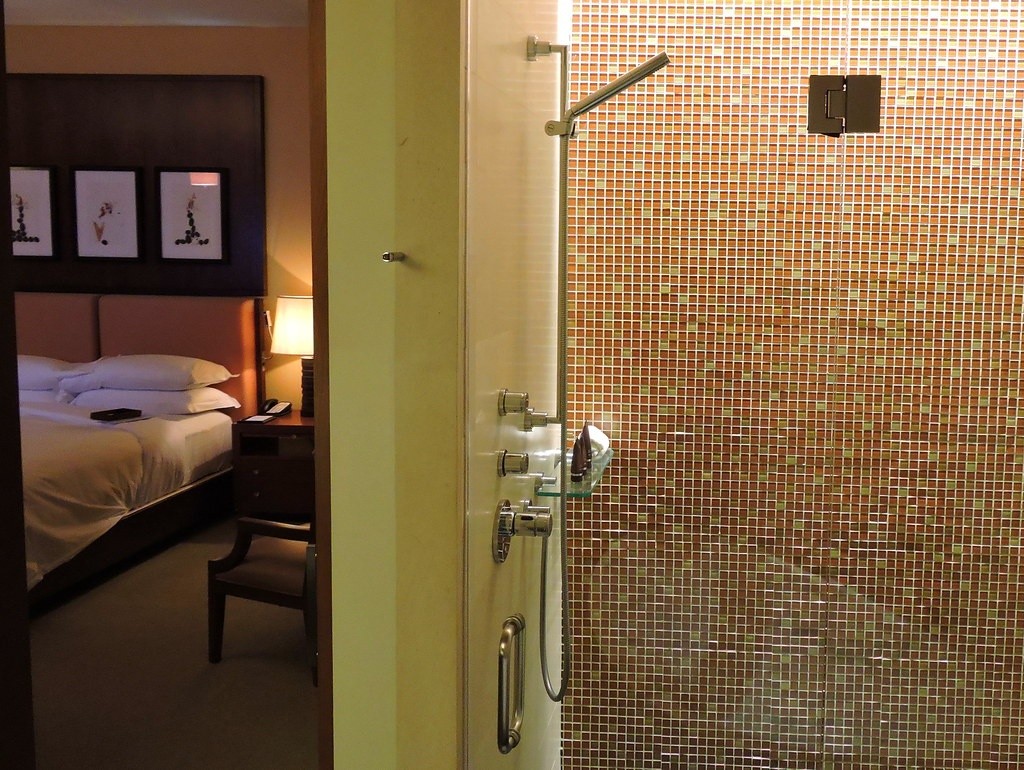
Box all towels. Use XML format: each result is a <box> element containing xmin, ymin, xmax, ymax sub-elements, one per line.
<box><xmin>577</xmin><ymin>425</ymin><xmax>610</xmax><ymax>461</ymax></box>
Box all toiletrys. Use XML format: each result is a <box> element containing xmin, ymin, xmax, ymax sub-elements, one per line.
<box><xmin>570</xmin><ymin>421</ymin><xmax>594</xmax><ymax>483</ymax></box>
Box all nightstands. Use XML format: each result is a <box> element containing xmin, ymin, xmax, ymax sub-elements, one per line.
<box><xmin>231</xmin><ymin>408</ymin><xmax>316</xmax><ymax>521</ymax></box>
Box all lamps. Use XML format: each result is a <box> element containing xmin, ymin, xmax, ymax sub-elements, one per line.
<box><xmin>270</xmin><ymin>295</ymin><xmax>315</xmax><ymax>418</ymax></box>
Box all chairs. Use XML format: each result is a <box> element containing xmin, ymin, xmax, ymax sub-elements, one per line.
<box><xmin>206</xmin><ymin>447</ymin><xmax>318</xmax><ymax>688</ymax></box>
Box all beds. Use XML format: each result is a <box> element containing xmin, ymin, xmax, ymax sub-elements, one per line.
<box><xmin>15</xmin><ymin>291</ymin><xmax>267</xmax><ymax>620</ymax></box>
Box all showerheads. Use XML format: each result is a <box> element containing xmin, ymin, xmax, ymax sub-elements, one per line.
<box><xmin>567</xmin><ymin>51</ymin><xmax>672</xmax><ymax>116</ymax></box>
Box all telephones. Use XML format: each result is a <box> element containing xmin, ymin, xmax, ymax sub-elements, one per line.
<box><xmin>260</xmin><ymin>398</ymin><xmax>292</xmax><ymax>416</ymax></box>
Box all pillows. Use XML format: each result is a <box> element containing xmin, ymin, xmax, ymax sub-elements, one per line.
<box><xmin>58</xmin><ymin>355</ymin><xmax>241</xmax><ymax>395</ymax></box>
<box><xmin>16</xmin><ymin>355</ymin><xmax>106</xmax><ymax>391</ymax></box>
<box><xmin>68</xmin><ymin>386</ymin><xmax>243</xmax><ymax>415</ymax></box>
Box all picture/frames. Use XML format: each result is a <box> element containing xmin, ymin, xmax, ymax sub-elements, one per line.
<box><xmin>69</xmin><ymin>164</ymin><xmax>146</xmax><ymax>264</ymax></box>
<box><xmin>152</xmin><ymin>166</ymin><xmax>230</xmax><ymax>264</ymax></box>
<box><xmin>0</xmin><ymin>162</ymin><xmax>63</xmax><ymax>262</ymax></box>
